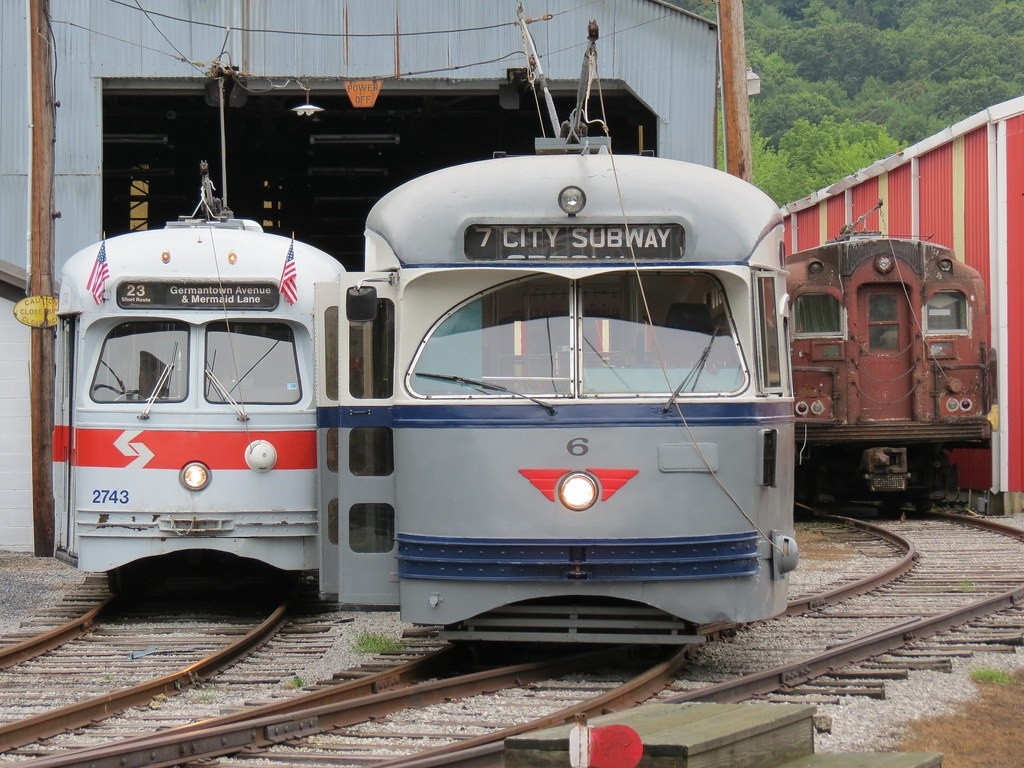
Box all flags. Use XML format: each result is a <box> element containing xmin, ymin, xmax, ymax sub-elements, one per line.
<box><xmin>278</xmin><ymin>241</ymin><xmax>298</xmax><ymax>306</ymax></box>
<box><xmin>87</xmin><ymin>240</ymin><xmax>110</xmax><ymax>306</ymax></box>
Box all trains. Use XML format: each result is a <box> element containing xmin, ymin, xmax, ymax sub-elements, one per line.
<box><xmin>312</xmin><ymin>152</ymin><xmax>798</xmax><ymax>657</ymax></box>
<box><xmin>51</xmin><ymin>159</ymin><xmax>352</xmax><ymax>606</ymax></box>
<box><xmin>783</xmin><ymin>239</ymin><xmax>1000</xmax><ymax>511</ymax></box>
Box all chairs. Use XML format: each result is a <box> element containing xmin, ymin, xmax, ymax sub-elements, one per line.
<box><xmin>664</xmin><ymin>302</ymin><xmax>718</xmax><ymax>363</ymax></box>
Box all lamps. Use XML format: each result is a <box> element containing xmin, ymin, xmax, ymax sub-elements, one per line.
<box><xmin>290</xmin><ymin>87</ymin><xmax>324</xmax><ymax>116</ymax></box>
